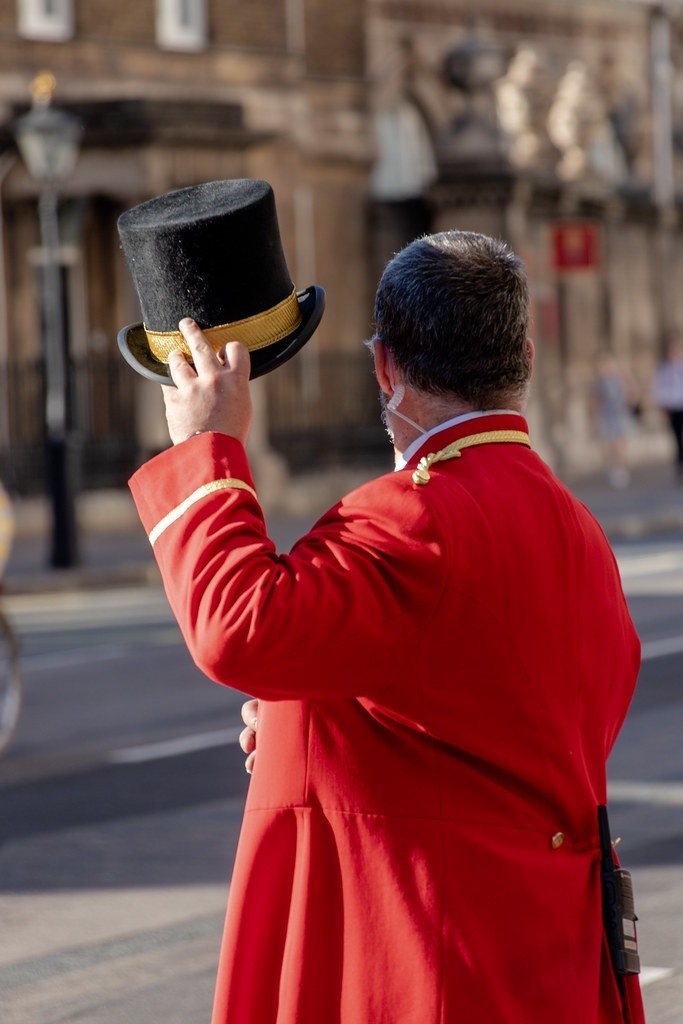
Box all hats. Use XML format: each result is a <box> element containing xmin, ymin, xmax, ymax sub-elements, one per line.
<box><xmin>110</xmin><ymin>179</ymin><xmax>327</xmax><ymax>388</ymax></box>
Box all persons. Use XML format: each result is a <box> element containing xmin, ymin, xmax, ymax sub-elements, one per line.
<box><xmin>127</xmin><ymin>230</ymin><xmax>642</xmax><ymax>1024</ymax></box>
<box><xmin>592</xmin><ymin>342</ymin><xmax>631</xmax><ymax>489</ymax></box>
<box><xmin>652</xmin><ymin>333</ymin><xmax>683</xmax><ymax>476</ymax></box>
